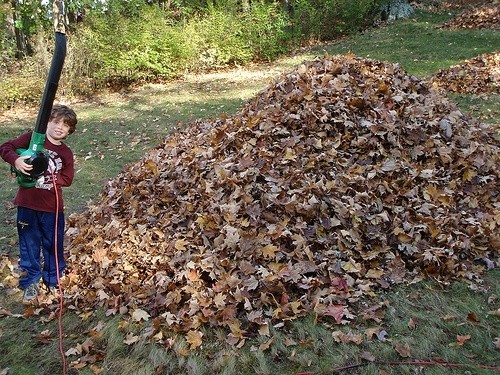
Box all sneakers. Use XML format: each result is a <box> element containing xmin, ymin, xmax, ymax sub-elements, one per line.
<box><xmin>22</xmin><ymin>283</ymin><xmax>40</xmax><ymax>304</ymax></box>
<box><xmin>46</xmin><ymin>285</ymin><xmax>61</xmax><ymax>295</ymax></box>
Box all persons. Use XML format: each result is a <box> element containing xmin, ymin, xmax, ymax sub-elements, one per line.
<box><xmin>0</xmin><ymin>103</ymin><xmax>78</xmax><ymax>306</ymax></box>
